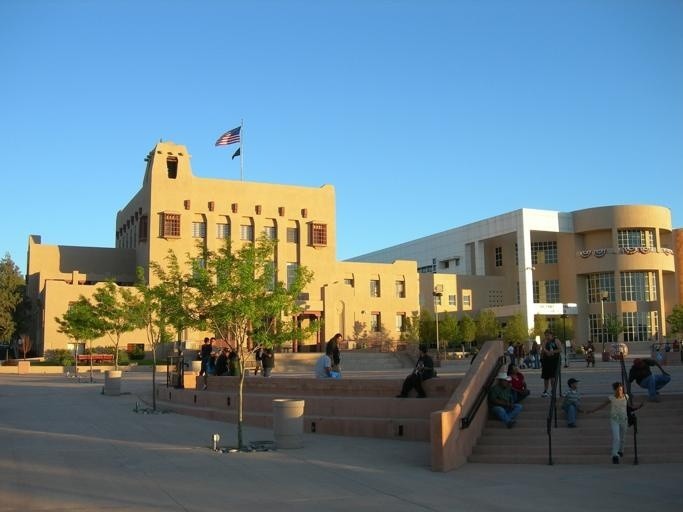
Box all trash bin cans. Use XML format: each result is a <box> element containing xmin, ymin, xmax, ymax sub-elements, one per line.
<box><xmin>184</xmin><ymin>360</ymin><xmax>202</xmax><ymax>376</ymax></box>
<box><xmin>104</xmin><ymin>371</ymin><xmax>122</xmax><ymax>396</ymax></box>
<box><xmin>272</xmin><ymin>399</ymin><xmax>305</xmax><ymax>449</ymax></box>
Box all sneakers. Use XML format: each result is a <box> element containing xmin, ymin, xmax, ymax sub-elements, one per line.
<box><xmin>568</xmin><ymin>423</ymin><xmax>576</xmax><ymax>427</ymax></box>
<box><xmin>395</xmin><ymin>392</ymin><xmax>427</xmax><ymax>398</ymax></box>
<box><xmin>612</xmin><ymin>451</ymin><xmax>623</xmax><ymax>464</ymax></box>
<box><xmin>507</xmin><ymin>420</ymin><xmax>516</xmax><ymax>429</ymax></box>
<box><xmin>541</xmin><ymin>391</ymin><xmax>552</xmax><ymax>397</ymax></box>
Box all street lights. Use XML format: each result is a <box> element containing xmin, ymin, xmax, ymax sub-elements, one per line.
<box><xmin>560</xmin><ymin>314</ymin><xmax>570</xmax><ymax>368</ymax></box>
<box><xmin>599</xmin><ymin>296</ymin><xmax>609</xmax><ymax>352</ymax></box>
<box><xmin>432</xmin><ymin>291</ymin><xmax>443</xmax><ymax>352</ymax></box>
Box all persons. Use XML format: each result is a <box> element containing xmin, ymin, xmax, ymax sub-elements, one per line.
<box><xmin>506</xmin><ymin>339</ymin><xmax>540</xmax><ymax>370</ymax></box>
<box><xmin>314</xmin><ymin>348</ymin><xmax>342</xmax><ymax>379</ymax></box>
<box><xmin>207</xmin><ymin>337</ymin><xmax>221</xmax><ymax>376</ymax></box>
<box><xmin>627</xmin><ymin>357</ymin><xmax>671</xmax><ymax>403</ymax></box>
<box><xmin>539</xmin><ymin>328</ymin><xmax>561</xmax><ymax>398</ymax></box>
<box><xmin>506</xmin><ymin>363</ymin><xmax>530</xmax><ymax>405</ymax></box>
<box><xmin>487</xmin><ymin>372</ymin><xmax>522</xmax><ymax>429</ymax></box>
<box><xmin>214</xmin><ymin>348</ymin><xmax>236</xmax><ymax>376</ymax></box>
<box><xmin>654</xmin><ymin>348</ymin><xmax>665</xmax><ymax>367</ymax></box>
<box><xmin>325</xmin><ymin>333</ymin><xmax>343</xmax><ymax>374</ymax></box>
<box><xmin>671</xmin><ymin>339</ymin><xmax>679</xmax><ymax>352</ymax></box>
<box><xmin>581</xmin><ymin>340</ymin><xmax>595</xmax><ymax>368</ymax></box>
<box><xmin>252</xmin><ymin>343</ymin><xmax>265</xmax><ymax>376</ymax></box>
<box><xmin>585</xmin><ymin>381</ymin><xmax>646</xmax><ymax>464</ymax></box>
<box><xmin>199</xmin><ymin>337</ymin><xmax>215</xmax><ymax>376</ymax></box>
<box><xmin>664</xmin><ymin>342</ymin><xmax>670</xmax><ymax>352</ymax></box>
<box><xmin>394</xmin><ymin>344</ymin><xmax>436</xmax><ymax>399</ymax></box>
<box><xmin>560</xmin><ymin>377</ymin><xmax>584</xmax><ymax>428</ymax></box>
<box><xmin>679</xmin><ymin>340</ymin><xmax>683</xmax><ymax>363</ymax></box>
<box><xmin>259</xmin><ymin>346</ymin><xmax>275</xmax><ymax>377</ymax></box>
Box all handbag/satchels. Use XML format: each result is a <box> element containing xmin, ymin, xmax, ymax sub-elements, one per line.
<box><xmin>625</xmin><ymin>393</ymin><xmax>632</xmax><ymax>416</ymax></box>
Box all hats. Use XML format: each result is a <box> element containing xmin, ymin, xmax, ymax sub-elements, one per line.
<box><xmin>567</xmin><ymin>378</ymin><xmax>579</xmax><ymax>385</ymax></box>
<box><xmin>494</xmin><ymin>372</ymin><xmax>513</xmax><ymax>381</ymax></box>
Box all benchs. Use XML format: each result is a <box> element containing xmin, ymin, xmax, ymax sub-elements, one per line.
<box><xmin>566</xmin><ymin>353</ymin><xmax>595</xmax><ymax>367</ymax></box>
<box><xmin>243</xmin><ymin>360</ymin><xmax>263</xmax><ymax>370</ymax></box>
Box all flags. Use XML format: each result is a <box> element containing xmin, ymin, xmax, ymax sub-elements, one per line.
<box><xmin>231</xmin><ymin>148</ymin><xmax>240</xmax><ymax>160</ymax></box>
<box><xmin>214</xmin><ymin>126</ymin><xmax>240</xmax><ymax>147</ymax></box>
